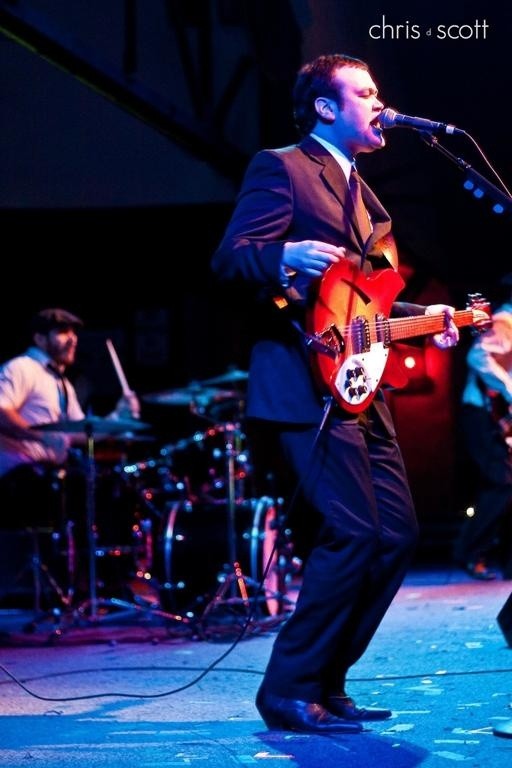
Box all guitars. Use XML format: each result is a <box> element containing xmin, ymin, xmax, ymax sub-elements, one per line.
<box><xmin>305</xmin><ymin>258</ymin><xmax>493</xmax><ymax>414</ymax></box>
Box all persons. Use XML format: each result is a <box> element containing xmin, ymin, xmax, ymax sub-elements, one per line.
<box><xmin>211</xmin><ymin>54</ymin><xmax>461</xmax><ymax>735</ymax></box>
<box><xmin>461</xmin><ymin>299</ymin><xmax>512</xmax><ymax>581</ymax></box>
<box><xmin>0</xmin><ymin>308</ymin><xmax>140</xmax><ymax>609</ymax></box>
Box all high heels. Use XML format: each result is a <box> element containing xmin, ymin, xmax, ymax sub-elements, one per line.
<box><xmin>456</xmin><ymin>558</ymin><xmax>496</xmax><ymax>579</ymax></box>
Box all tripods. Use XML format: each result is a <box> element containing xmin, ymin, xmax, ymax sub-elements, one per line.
<box><xmin>22</xmin><ymin>414</ymin><xmax>294</xmax><ymax>645</ymax></box>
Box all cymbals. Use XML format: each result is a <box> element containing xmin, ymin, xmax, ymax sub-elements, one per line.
<box><xmin>29</xmin><ymin>418</ymin><xmax>146</xmax><ymax>435</ymax></box>
<box><xmin>203</xmin><ymin>373</ymin><xmax>252</xmax><ymax>384</ymax></box>
<box><xmin>141</xmin><ymin>390</ymin><xmax>237</xmax><ymax>405</ymax></box>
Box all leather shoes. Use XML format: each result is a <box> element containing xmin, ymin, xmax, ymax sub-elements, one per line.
<box><xmin>254</xmin><ymin>694</ymin><xmax>392</xmax><ymax>733</ymax></box>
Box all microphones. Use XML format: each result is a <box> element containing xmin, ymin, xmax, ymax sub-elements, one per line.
<box><xmin>379</xmin><ymin>108</ymin><xmax>466</xmax><ymax>135</ymax></box>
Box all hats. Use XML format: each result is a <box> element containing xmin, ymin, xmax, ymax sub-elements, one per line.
<box><xmin>33</xmin><ymin>307</ymin><xmax>86</xmax><ymax>332</ymax></box>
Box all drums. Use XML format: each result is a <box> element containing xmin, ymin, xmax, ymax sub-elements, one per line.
<box><xmin>119</xmin><ymin>461</ymin><xmax>184</xmax><ymax>504</ymax></box>
<box><xmin>166</xmin><ymin>499</ymin><xmax>286</xmax><ymax>624</ymax></box>
<box><xmin>164</xmin><ymin>419</ymin><xmax>253</xmax><ymax>494</ymax></box>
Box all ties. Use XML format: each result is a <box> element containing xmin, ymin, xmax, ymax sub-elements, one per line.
<box><xmin>344</xmin><ymin>167</ymin><xmax>373</xmax><ymax>253</ymax></box>
